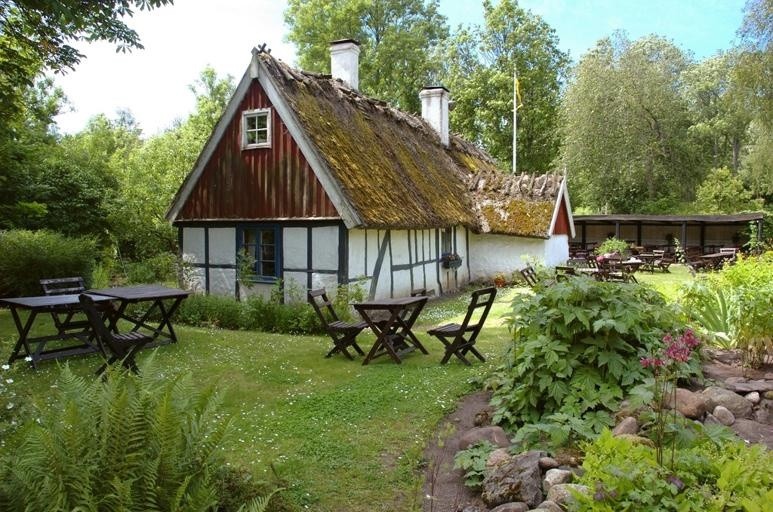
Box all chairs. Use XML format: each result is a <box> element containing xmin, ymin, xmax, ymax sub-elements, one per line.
<box><xmin>39</xmin><ymin>275</ymin><xmax>152</xmax><ymax>385</ymax></box>
<box><xmin>520</xmin><ymin>240</ymin><xmax>741</xmax><ymax>291</ymax></box>
<box><xmin>427</xmin><ymin>287</ymin><xmax>497</xmax><ymax>367</ymax></box>
<box><xmin>307</xmin><ymin>288</ymin><xmax>370</xmax><ymax>361</ymax></box>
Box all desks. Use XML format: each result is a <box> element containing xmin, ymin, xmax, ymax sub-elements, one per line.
<box><xmin>0</xmin><ymin>285</ymin><xmax>188</xmax><ymax>373</ymax></box>
<box><xmin>348</xmin><ymin>296</ymin><xmax>429</xmax><ymax>366</ymax></box>
